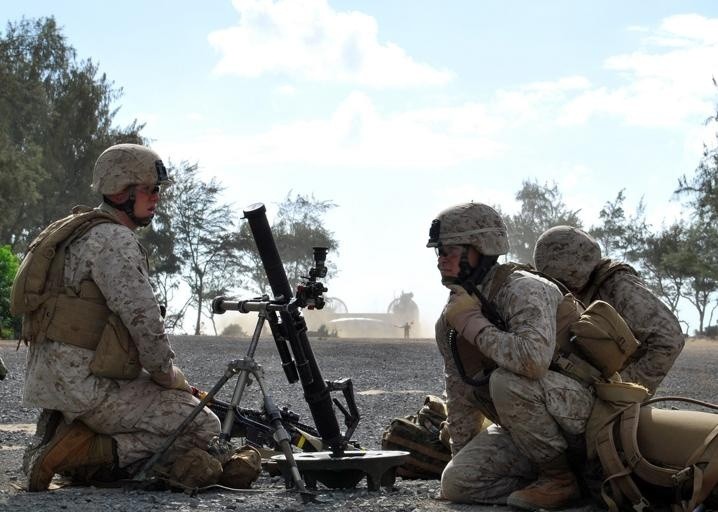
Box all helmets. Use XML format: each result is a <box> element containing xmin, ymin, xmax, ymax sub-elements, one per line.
<box><xmin>533</xmin><ymin>225</ymin><xmax>601</xmax><ymax>288</ymax></box>
<box><xmin>93</xmin><ymin>143</ymin><xmax>169</xmax><ymax>195</ymax></box>
<box><xmin>426</xmin><ymin>200</ymin><xmax>510</xmax><ymax>255</ymax></box>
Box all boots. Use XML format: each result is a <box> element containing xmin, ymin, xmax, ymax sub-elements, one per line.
<box><xmin>506</xmin><ymin>448</ymin><xmax>581</xmax><ymax>512</ymax></box>
<box><xmin>23</xmin><ymin>409</ymin><xmax>114</xmax><ymax>492</ymax></box>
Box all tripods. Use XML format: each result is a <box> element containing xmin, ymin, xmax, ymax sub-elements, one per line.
<box><xmin>120</xmin><ymin>293</ymin><xmax>319</xmax><ymax>505</ymax></box>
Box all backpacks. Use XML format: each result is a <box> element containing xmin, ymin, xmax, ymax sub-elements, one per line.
<box><xmin>587</xmin><ymin>397</ymin><xmax>718</xmax><ymax>512</ymax></box>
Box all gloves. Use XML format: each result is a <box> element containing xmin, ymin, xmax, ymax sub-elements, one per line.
<box><xmin>150</xmin><ymin>366</ymin><xmax>194</xmax><ymax>394</ymax></box>
<box><xmin>443</xmin><ymin>284</ymin><xmax>495</xmax><ymax>346</ymax></box>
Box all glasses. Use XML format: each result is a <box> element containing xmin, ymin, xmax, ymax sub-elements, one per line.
<box><xmin>438</xmin><ymin>246</ymin><xmax>457</xmax><ymax>257</ymax></box>
<box><xmin>133</xmin><ymin>184</ymin><xmax>160</xmax><ymax>196</ymax></box>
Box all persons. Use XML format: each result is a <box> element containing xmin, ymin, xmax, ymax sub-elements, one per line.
<box><xmin>426</xmin><ymin>199</ymin><xmax>626</xmax><ymax>510</ymax></box>
<box><xmin>529</xmin><ymin>224</ymin><xmax>689</xmax><ymax>418</ymax></box>
<box><xmin>9</xmin><ymin>142</ymin><xmax>223</xmax><ymax>495</ymax></box>
